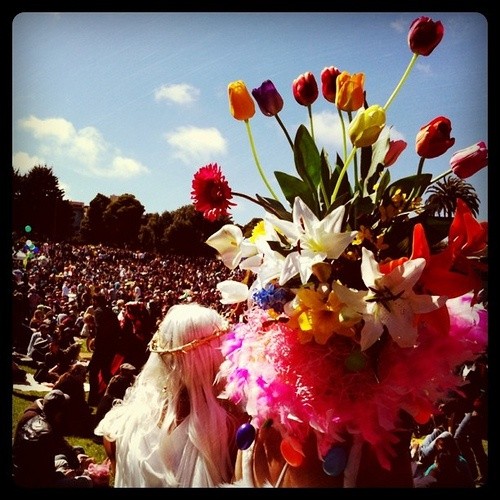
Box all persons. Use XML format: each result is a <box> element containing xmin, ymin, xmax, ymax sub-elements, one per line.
<box><xmin>4</xmin><ymin>234</ymin><xmax>494</xmax><ymax>492</ymax></box>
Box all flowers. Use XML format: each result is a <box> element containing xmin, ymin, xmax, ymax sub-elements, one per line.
<box><xmin>191</xmin><ymin>13</ymin><xmax>487</xmax><ymax>488</ymax></box>
